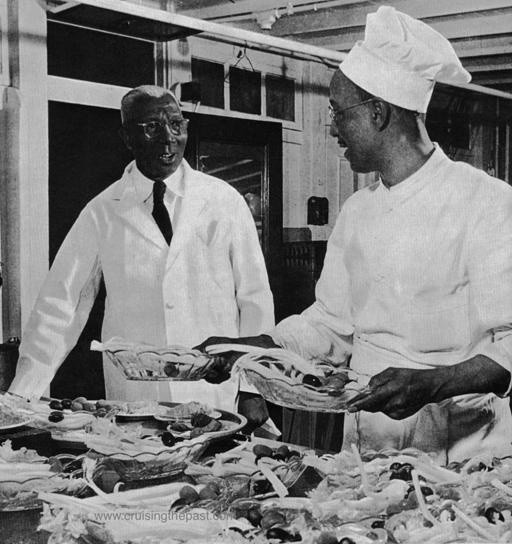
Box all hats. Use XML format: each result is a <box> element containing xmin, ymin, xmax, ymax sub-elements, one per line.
<box><xmin>338</xmin><ymin>5</ymin><xmax>472</xmax><ymax>113</ymax></box>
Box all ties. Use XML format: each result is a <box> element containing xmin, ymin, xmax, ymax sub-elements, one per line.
<box><xmin>152</xmin><ymin>180</ymin><xmax>173</xmax><ymax>246</ymax></box>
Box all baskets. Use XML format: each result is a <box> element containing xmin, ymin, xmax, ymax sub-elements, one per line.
<box><xmin>240</xmin><ymin>356</ymin><xmax>373</xmax><ymax>415</ymax></box>
<box><xmin>1</xmin><ymin>395</ymin><xmax>512</xmax><ymax>543</ymax></box>
<box><xmin>88</xmin><ymin>336</ymin><xmax>219</xmax><ymax>382</ymax></box>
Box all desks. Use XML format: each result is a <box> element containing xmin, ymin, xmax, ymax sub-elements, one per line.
<box><xmin>0</xmin><ymin>393</ymin><xmax>392</xmax><ymax>544</ymax></box>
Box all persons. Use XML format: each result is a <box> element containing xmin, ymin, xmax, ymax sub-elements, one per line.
<box><xmin>191</xmin><ymin>4</ymin><xmax>512</xmax><ymax>468</ymax></box>
<box><xmin>6</xmin><ymin>87</ymin><xmax>276</xmax><ymax>435</ymax></box>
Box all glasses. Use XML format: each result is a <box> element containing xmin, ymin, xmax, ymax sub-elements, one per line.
<box><xmin>328</xmin><ymin>97</ymin><xmax>385</xmax><ymax>121</ymax></box>
<box><xmin>135</xmin><ymin>119</ymin><xmax>190</xmax><ymax>139</ymax></box>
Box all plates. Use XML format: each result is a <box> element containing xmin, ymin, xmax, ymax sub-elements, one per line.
<box><xmin>89</xmin><ymin>399</ymin><xmax>154</xmax><ymax>420</ymax></box>
<box><xmin>158</xmin><ymin>400</ymin><xmax>247</xmax><ymax>444</ymax></box>
<box><xmin>0</xmin><ymin>414</ymin><xmax>31</xmax><ymax>430</ymax></box>
